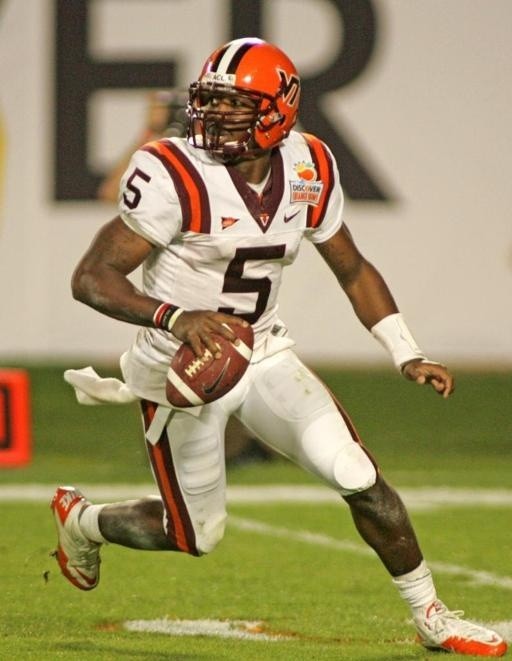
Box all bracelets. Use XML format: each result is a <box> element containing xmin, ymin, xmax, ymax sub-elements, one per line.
<box><xmin>368</xmin><ymin>310</ymin><xmax>428</xmax><ymax>371</ymax></box>
<box><xmin>149</xmin><ymin>297</ymin><xmax>182</xmax><ymax>334</ymax></box>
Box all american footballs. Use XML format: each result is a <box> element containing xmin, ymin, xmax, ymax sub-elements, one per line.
<box><xmin>166</xmin><ymin>320</ymin><xmax>254</xmax><ymax>408</ymax></box>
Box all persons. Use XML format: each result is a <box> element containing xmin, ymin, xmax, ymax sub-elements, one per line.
<box><xmin>48</xmin><ymin>32</ymin><xmax>508</xmax><ymax>657</ymax></box>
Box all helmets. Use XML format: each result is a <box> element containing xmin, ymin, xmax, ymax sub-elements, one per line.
<box><xmin>184</xmin><ymin>36</ymin><xmax>301</xmax><ymax>166</ymax></box>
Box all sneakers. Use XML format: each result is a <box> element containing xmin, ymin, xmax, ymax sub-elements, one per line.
<box><xmin>49</xmin><ymin>485</ymin><xmax>101</xmax><ymax>589</ymax></box>
<box><xmin>414</xmin><ymin>598</ymin><xmax>508</xmax><ymax>657</ymax></box>
